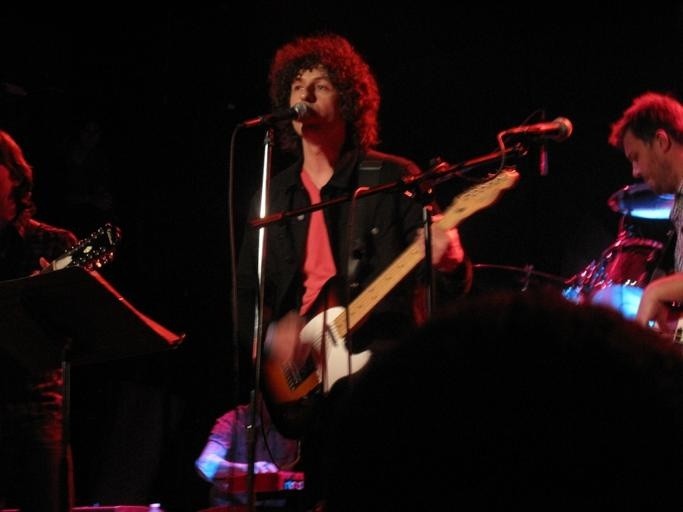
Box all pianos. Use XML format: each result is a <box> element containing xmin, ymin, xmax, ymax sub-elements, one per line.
<box><xmin>216</xmin><ymin>469</ymin><xmax>306</xmax><ymax>496</ymax></box>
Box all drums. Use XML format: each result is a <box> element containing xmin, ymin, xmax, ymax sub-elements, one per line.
<box><xmin>575</xmin><ymin>237</ymin><xmax>683</xmax><ymax>344</ymax></box>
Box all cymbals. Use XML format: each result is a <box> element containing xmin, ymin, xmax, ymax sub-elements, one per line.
<box><xmin>608</xmin><ymin>182</ymin><xmax>676</xmax><ymax>220</ymax></box>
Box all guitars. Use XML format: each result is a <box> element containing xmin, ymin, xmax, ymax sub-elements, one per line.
<box><xmin>1</xmin><ymin>224</ymin><xmax>122</xmax><ymax>286</ymax></box>
<box><xmin>262</xmin><ymin>166</ymin><xmax>519</xmax><ymax>442</ymax></box>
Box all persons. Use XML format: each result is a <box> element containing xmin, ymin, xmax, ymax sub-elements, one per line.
<box><xmin>237</xmin><ymin>33</ymin><xmax>474</xmax><ymax>511</ymax></box>
<box><xmin>0</xmin><ymin>132</ymin><xmax>106</xmax><ymax>512</ymax></box>
<box><xmin>193</xmin><ymin>386</ymin><xmax>301</xmax><ymax>512</ymax></box>
<box><xmin>606</xmin><ymin>93</ymin><xmax>681</xmax><ymax>346</ymax></box>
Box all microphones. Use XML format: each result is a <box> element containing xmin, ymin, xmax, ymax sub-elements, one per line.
<box><xmin>242</xmin><ymin>102</ymin><xmax>307</xmax><ymax>129</ymax></box>
<box><xmin>499</xmin><ymin>115</ymin><xmax>574</xmax><ymax>141</ymax></box>
